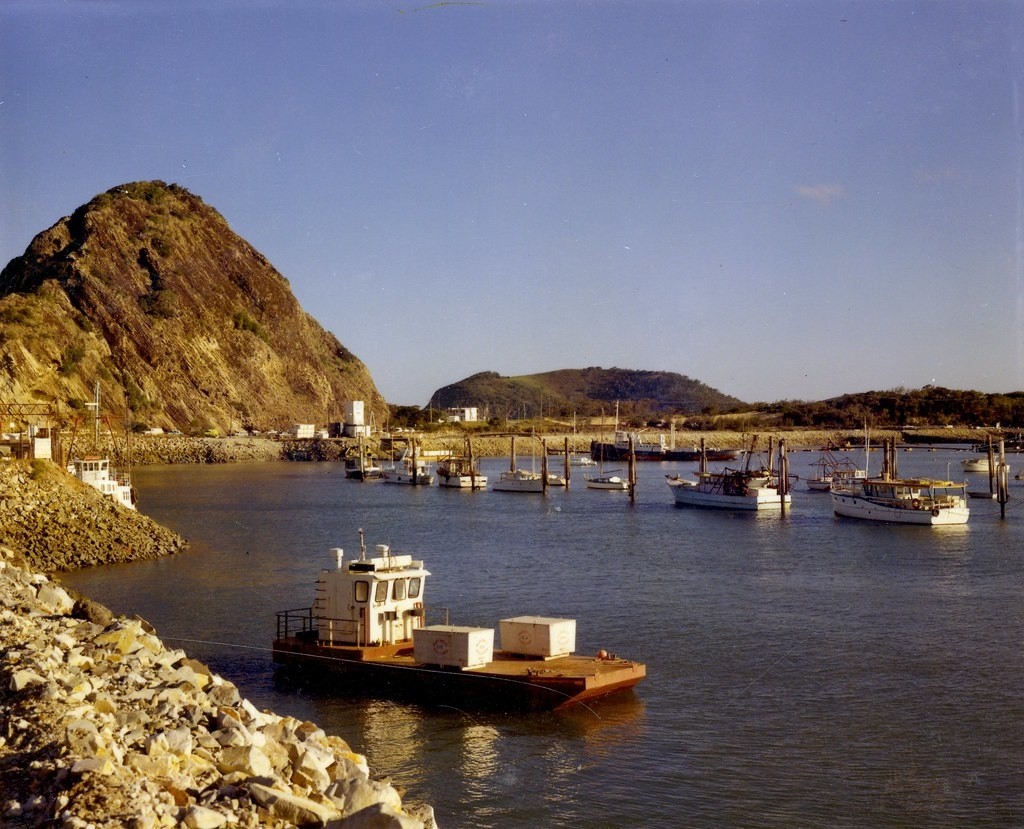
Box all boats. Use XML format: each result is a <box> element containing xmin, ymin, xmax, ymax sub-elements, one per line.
<box><xmin>65</xmin><ymin>379</ymin><xmax>138</xmax><ymax>512</ymax></box>
<box><xmin>806</xmin><ymin>447</ymin><xmax>866</xmax><ymax>490</ymax></box>
<box><xmin>590</xmin><ymin>399</ymin><xmax>741</xmax><ymax>461</ymax></box>
<box><xmin>664</xmin><ymin>434</ymin><xmax>798</xmax><ymax>505</ymax></box>
<box><xmin>337</xmin><ymin>435</ymin><xmax>379</xmax><ymax>478</ymax></box>
<box><xmin>493</xmin><ymin>436</ymin><xmax>566</xmax><ymax>492</ymax></box>
<box><xmin>960</xmin><ymin>455</ymin><xmax>1010</xmax><ymax>472</ymax></box>
<box><xmin>829</xmin><ymin>418</ymin><xmax>970</xmax><ymax>524</ymax></box>
<box><xmin>435</xmin><ymin>430</ymin><xmax>488</xmax><ymax>487</ymax></box>
<box><xmin>379</xmin><ymin>437</ymin><xmax>434</xmax><ymax>484</ymax></box>
<box><xmin>584</xmin><ymin>408</ymin><xmax>629</xmax><ymax>489</ymax></box>
<box><xmin>272</xmin><ymin>527</ymin><xmax>645</xmax><ymax>712</ymax></box>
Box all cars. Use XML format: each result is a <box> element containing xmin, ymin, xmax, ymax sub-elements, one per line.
<box><xmin>168</xmin><ymin>430</ymin><xmax>184</xmax><ymax>436</ymax></box>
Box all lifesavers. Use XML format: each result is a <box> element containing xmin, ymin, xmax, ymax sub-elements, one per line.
<box><xmin>932</xmin><ymin>508</ymin><xmax>940</xmax><ymax>516</ymax></box>
<box><xmin>912</xmin><ymin>498</ymin><xmax>919</xmax><ymax>507</ymax></box>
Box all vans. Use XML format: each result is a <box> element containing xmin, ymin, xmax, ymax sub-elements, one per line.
<box><xmin>235</xmin><ymin>431</ymin><xmax>248</xmax><ymax>437</ymax></box>
